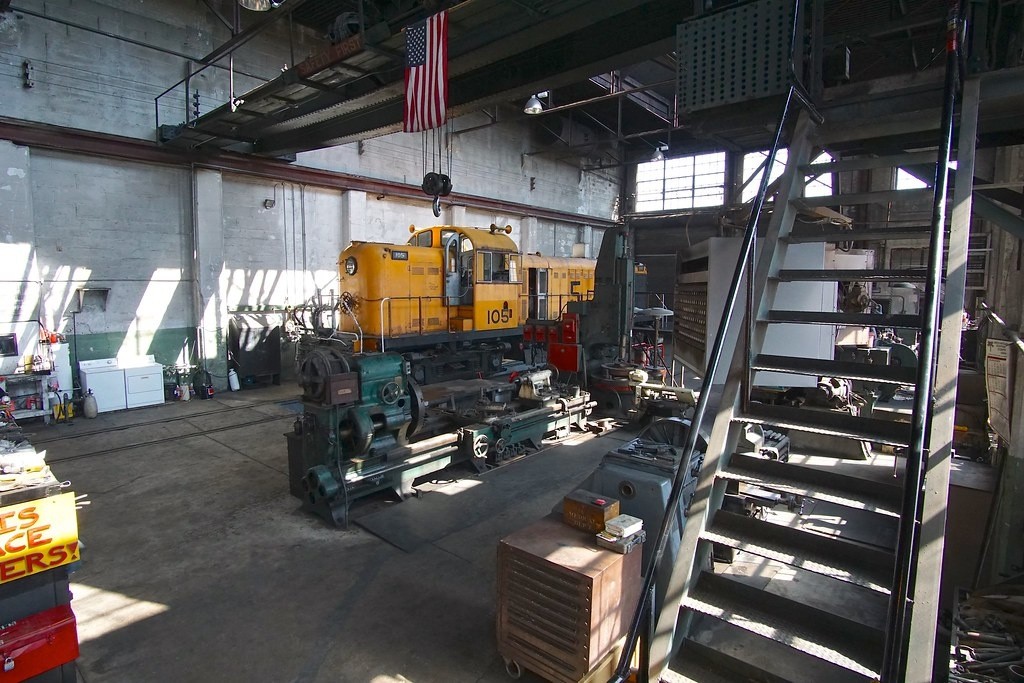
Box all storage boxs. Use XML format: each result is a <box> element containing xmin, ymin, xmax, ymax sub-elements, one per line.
<box><xmin>597</xmin><ymin>530</ymin><xmax>647</xmax><ymax>553</ymax></box>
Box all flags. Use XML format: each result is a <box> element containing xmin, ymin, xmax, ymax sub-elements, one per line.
<box><xmin>403</xmin><ymin>8</ymin><xmax>449</xmax><ymax>133</ymax></box>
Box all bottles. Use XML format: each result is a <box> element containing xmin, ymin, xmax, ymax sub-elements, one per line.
<box><xmin>294</xmin><ymin>417</ymin><xmax>302</xmax><ymax>434</ymax></box>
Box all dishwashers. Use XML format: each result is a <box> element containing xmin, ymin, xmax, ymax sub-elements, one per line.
<box><xmin>117</xmin><ymin>355</ymin><xmax>165</xmax><ymax>408</ymax></box>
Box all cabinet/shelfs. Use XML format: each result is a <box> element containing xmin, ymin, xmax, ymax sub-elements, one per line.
<box><xmin>0</xmin><ymin>373</ymin><xmax>53</xmax><ymax>424</ymax></box>
<box><xmin>120</xmin><ymin>353</ymin><xmax>166</xmax><ymax>407</ymax></box>
<box><xmin>497</xmin><ymin>511</ymin><xmax>644</xmax><ymax>682</ymax></box>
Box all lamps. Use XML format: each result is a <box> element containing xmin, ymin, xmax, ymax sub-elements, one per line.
<box><xmin>651</xmin><ymin>147</ymin><xmax>664</xmax><ymax>162</ymax></box>
<box><xmin>238</xmin><ymin>0</ymin><xmax>271</xmax><ymax>12</ymax></box>
<box><xmin>524</xmin><ymin>95</ymin><xmax>543</xmax><ymax>115</ymax></box>
